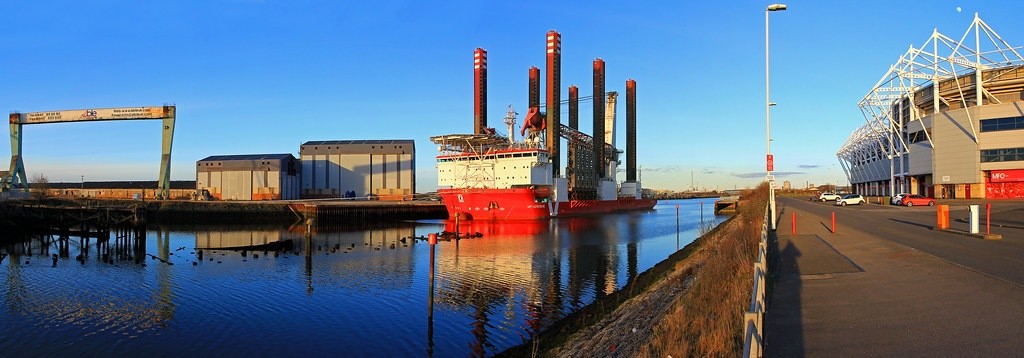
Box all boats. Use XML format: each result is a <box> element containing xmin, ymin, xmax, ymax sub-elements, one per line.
<box><xmin>429</xmin><ymin>29</ymin><xmax>659</xmax><ymax>222</ymax></box>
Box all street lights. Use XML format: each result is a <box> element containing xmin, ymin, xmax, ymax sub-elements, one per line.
<box><xmin>765</xmin><ymin>3</ymin><xmax>788</xmax><ymax>232</ymax></box>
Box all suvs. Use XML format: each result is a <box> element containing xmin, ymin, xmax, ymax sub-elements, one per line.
<box><xmin>819</xmin><ymin>193</ymin><xmax>842</xmax><ymax>202</ymax></box>
<box><xmin>901</xmin><ymin>193</ymin><xmax>936</xmax><ymax>207</ymax></box>
<box><xmin>836</xmin><ymin>193</ymin><xmax>865</xmax><ymax>207</ymax></box>
<box><xmin>892</xmin><ymin>193</ymin><xmax>909</xmax><ymax>206</ymax></box>
